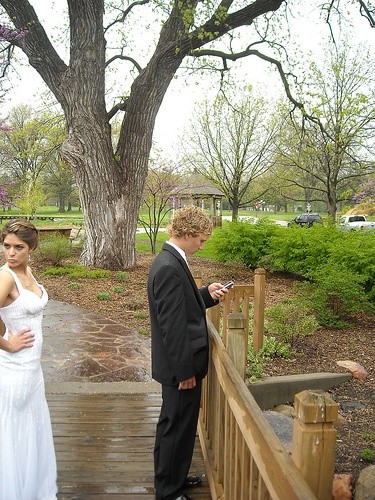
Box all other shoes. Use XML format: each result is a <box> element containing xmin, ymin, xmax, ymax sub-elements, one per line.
<box><xmin>176</xmin><ymin>493</ymin><xmax>192</xmax><ymax>500</ymax></box>
<box><xmin>184</xmin><ymin>474</ymin><xmax>202</xmax><ymax>488</ymax></box>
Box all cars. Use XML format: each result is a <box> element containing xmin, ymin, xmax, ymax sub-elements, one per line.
<box><xmin>287</xmin><ymin>213</ymin><xmax>322</xmax><ymax>227</ymax></box>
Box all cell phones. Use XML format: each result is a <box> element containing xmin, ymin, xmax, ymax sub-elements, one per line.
<box><xmin>219</xmin><ymin>281</ymin><xmax>234</xmax><ymax>291</ymax></box>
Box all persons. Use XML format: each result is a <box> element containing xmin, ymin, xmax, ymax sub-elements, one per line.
<box><xmin>147</xmin><ymin>206</ymin><xmax>228</xmax><ymax>500</ymax></box>
<box><xmin>0</xmin><ymin>218</ymin><xmax>59</xmax><ymax>499</ymax></box>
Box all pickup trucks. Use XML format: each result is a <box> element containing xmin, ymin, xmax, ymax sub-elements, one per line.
<box><xmin>336</xmin><ymin>215</ymin><xmax>375</xmax><ymax>231</ymax></box>
